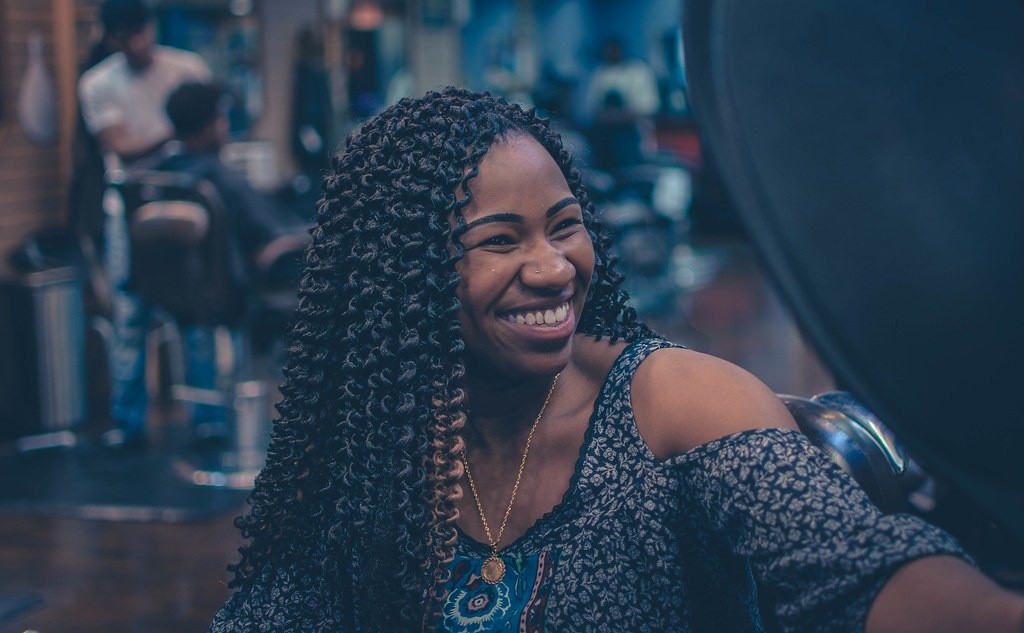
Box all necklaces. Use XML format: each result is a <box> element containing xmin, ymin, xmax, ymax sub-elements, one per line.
<box><xmin>458</xmin><ymin>371</ymin><xmax>559</xmax><ymax>584</ymax></box>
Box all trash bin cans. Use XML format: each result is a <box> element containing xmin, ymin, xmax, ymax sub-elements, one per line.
<box><xmin>0</xmin><ymin>256</ymin><xmax>91</xmax><ymax>433</ymax></box>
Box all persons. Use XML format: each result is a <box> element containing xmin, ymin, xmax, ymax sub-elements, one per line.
<box><xmin>209</xmin><ymin>89</ymin><xmax>1024</xmax><ymax>633</ymax></box>
<box><xmin>80</xmin><ymin>0</ymin><xmax>314</xmax><ymax>463</ymax></box>
<box><xmin>478</xmin><ymin>32</ymin><xmax>660</xmax><ymax>196</ymax></box>
